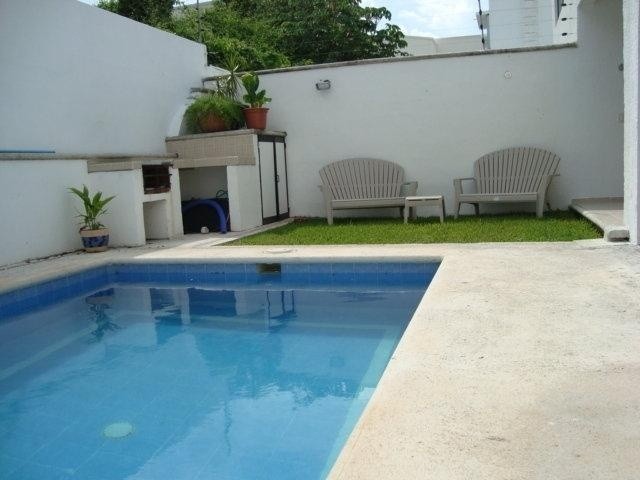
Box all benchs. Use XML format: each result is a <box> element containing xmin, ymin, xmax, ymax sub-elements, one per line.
<box><xmin>454</xmin><ymin>147</ymin><xmax>561</xmax><ymax>220</ymax></box>
<box><xmin>318</xmin><ymin>158</ymin><xmax>417</xmax><ymax>225</ymax></box>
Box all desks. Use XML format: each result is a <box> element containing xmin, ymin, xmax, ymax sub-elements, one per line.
<box><xmin>403</xmin><ymin>196</ymin><xmax>444</xmax><ymax>224</ymax></box>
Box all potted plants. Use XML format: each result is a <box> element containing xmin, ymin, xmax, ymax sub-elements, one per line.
<box><xmin>66</xmin><ymin>185</ymin><xmax>117</xmax><ymax>252</ymax></box>
<box><xmin>85</xmin><ymin>288</ymin><xmax>124</xmax><ymax>345</ymax></box>
<box><xmin>242</xmin><ymin>71</ymin><xmax>272</xmax><ymax>129</ymax></box>
<box><xmin>186</xmin><ymin>90</ymin><xmax>244</xmax><ymax>133</ymax></box>
<box><xmin>215</xmin><ymin>37</ymin><xmax>250</xmax><ymax>130</ymax></box>
